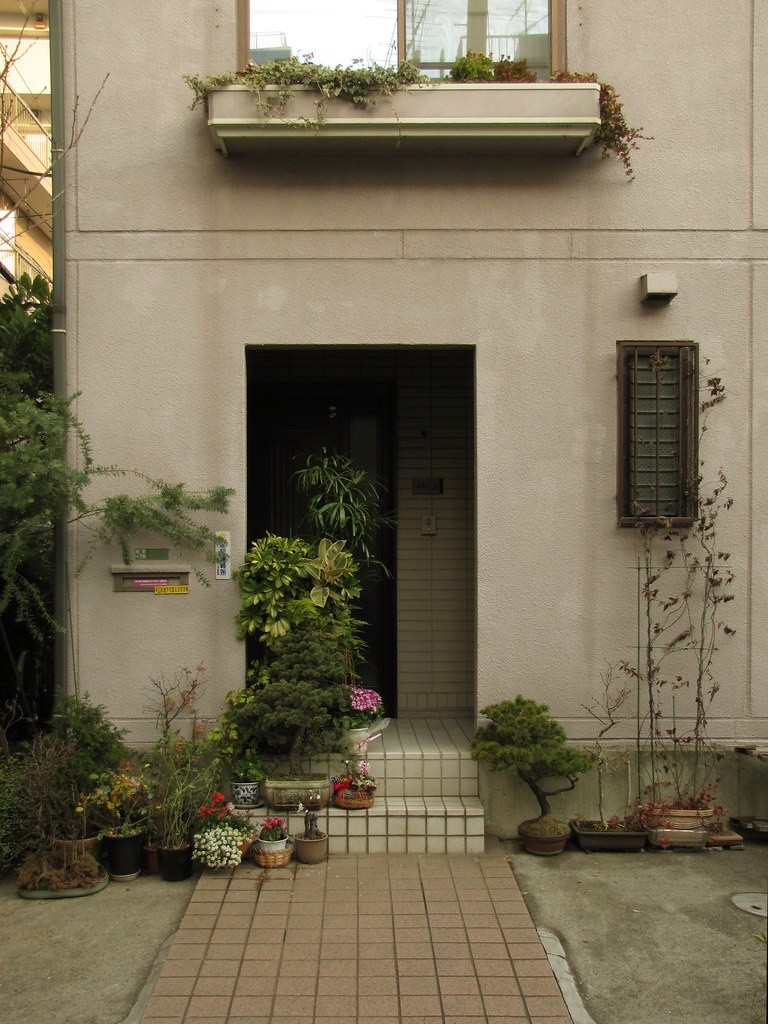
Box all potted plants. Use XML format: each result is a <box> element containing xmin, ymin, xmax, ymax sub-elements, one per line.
<box><xmin>294</xmin><ymin>813</ymin><xmax>329</xmax><ymax>865</ymax></box>
<box><xmin>146</xmin><ymin>750</ymin><xmax>216</xmax><ymax>881</ymax></box>
<box><xmin>238</xmin><ymin>651</ymin><xmax>355</xmax><ymax>807</ymax></box>
<box><xmin>571</xmin><ymin>460</ymin><xmax>736</xmax><ymax>850</ymax></box>
<box><xmin>16</xmin><ymin>732</ymin><xmax>116</xmax><ymax>898</ymax></box>
<box><xmin>471</xmin><ymin>697</ymin><xmax>602</xmax><ymax>858</ymax></box>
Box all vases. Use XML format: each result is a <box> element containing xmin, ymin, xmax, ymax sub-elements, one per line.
<box><xmin>104</xmin><ymin>834</ymin><xmax>146</xmax><ymax>882</ymax></box>
<box><xmin>336</xmin><ymin>790</ymin><xmax>376</xmax><ymax>808</ymax></box>
<box><xmin>256</xmin><ymin>835</ymin><xmax>290</xmax><ymax>852</ymax></box>
<box><xmin>210</xmin><ymin>833</ymin><xmax>255</xmax><ymax>868</ymax></box>
<box><xmin>348</xmin><ymin>728</ymin><xmax>370</xmax><ymax>755</ymax></box>
<box><xmin>231</xmin><ymin>781</ymin><xmax>257</xmax><ymax>805</ymax></box>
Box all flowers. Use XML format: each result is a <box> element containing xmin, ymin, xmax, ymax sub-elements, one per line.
<box><xmin>258</xmin><ymin>816</ymin><xmax>287</xmax><ymax>841</ymax></box>
<box><xmin>345</xmin><ymin>689</ymin><xmax>382</xmax><ymax>728</ymax></box>
<box><xmin>331</xmin><ymin>759</ymin><xmax>378</xmax><ymax>790</ymax></box>
<box><xmin>192</xmin><ymin>793</ymin><xmax>258</xmax><ymax>872</ymax></box>
<box><xmin>234</xmin><ymin>761</ymin><xmax>267</xmax><ymax>786</ymax></box>
<box><xmin>77</xmin><ymin>774</ymin><xmax>164</xmax><ymax>837</ymax></box>
